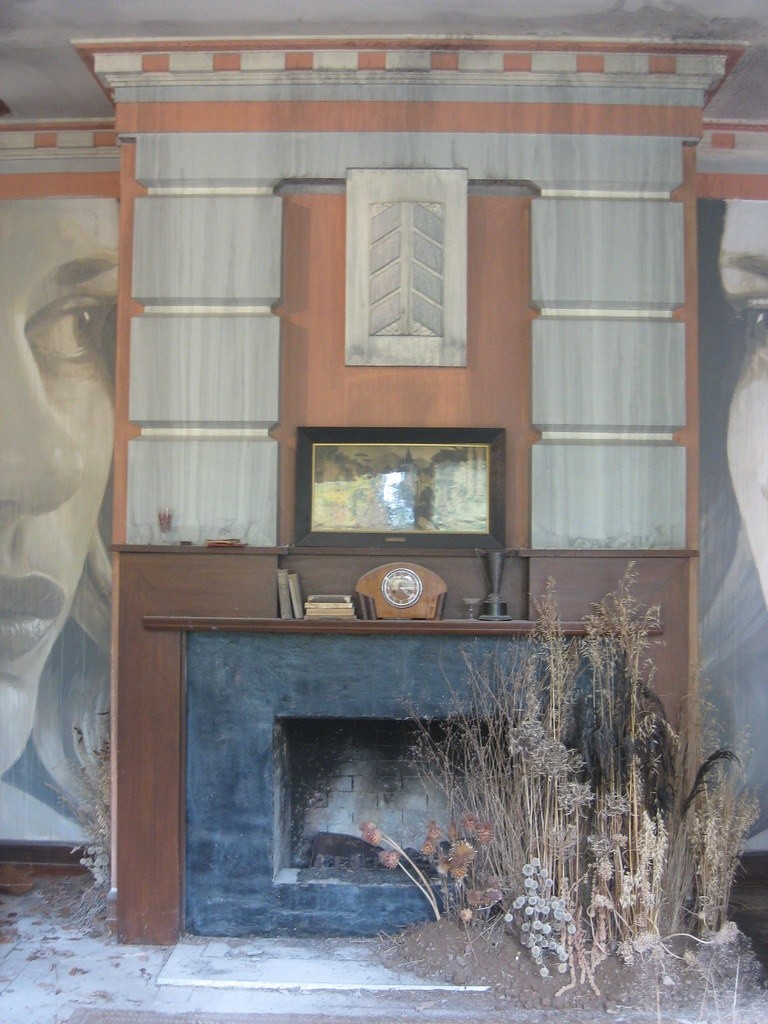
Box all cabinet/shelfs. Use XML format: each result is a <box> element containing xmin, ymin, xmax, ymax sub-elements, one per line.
<box><xmin>107</xmin><ymin>543</ymin><xmax>700</xmax><ymax>944</ymax></box>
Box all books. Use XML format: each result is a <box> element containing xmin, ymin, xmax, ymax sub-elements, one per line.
<box><xmin>275</xmin><ymin>566</ymin><xmax>359</xmax><ymax>622</ymax></box>
<box><xmin>205</xmin><ymin>538</ymin><xmax>249</xmax><ymax>547</ymax></box>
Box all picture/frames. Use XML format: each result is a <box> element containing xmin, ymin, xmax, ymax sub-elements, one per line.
<box><xmin>294</xmin><ymin>426</ymin><xmax>506</xmax><ymax>550</ymax></box>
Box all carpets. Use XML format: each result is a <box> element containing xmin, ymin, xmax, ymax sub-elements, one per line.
<box><xmin>156</xmin><ymin>933</ymin><xmax>491</xmax><ymax>992</ymax></box>
